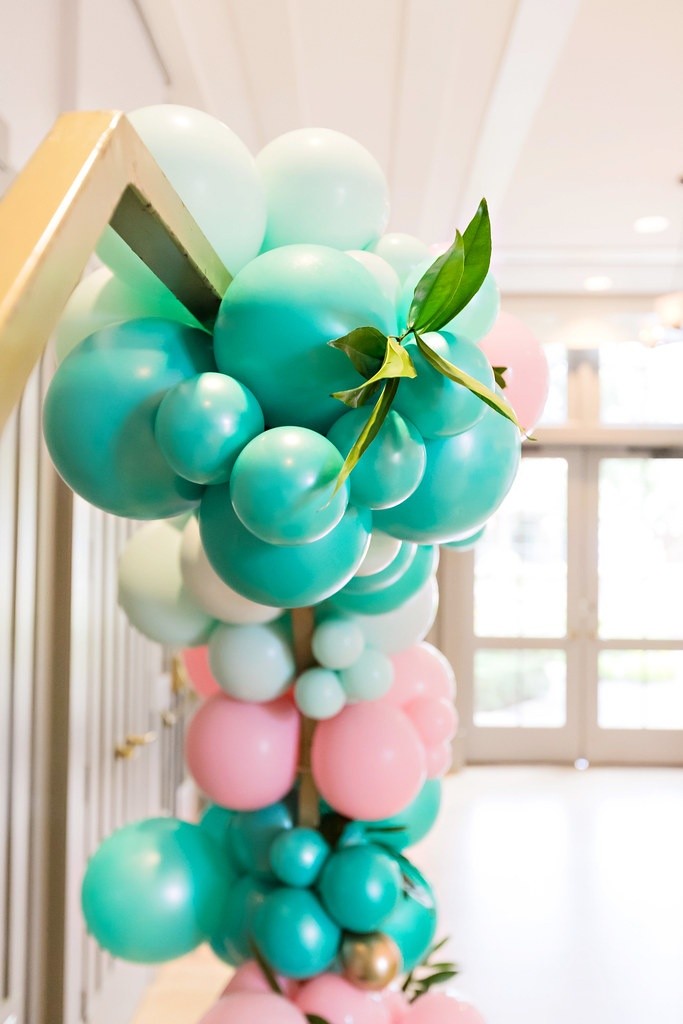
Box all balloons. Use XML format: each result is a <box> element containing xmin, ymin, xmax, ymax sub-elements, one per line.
<box><xmin>42</xmin><ymin>103</ymin><xmax>549</xmax><ymax>1024</ymax></box>
